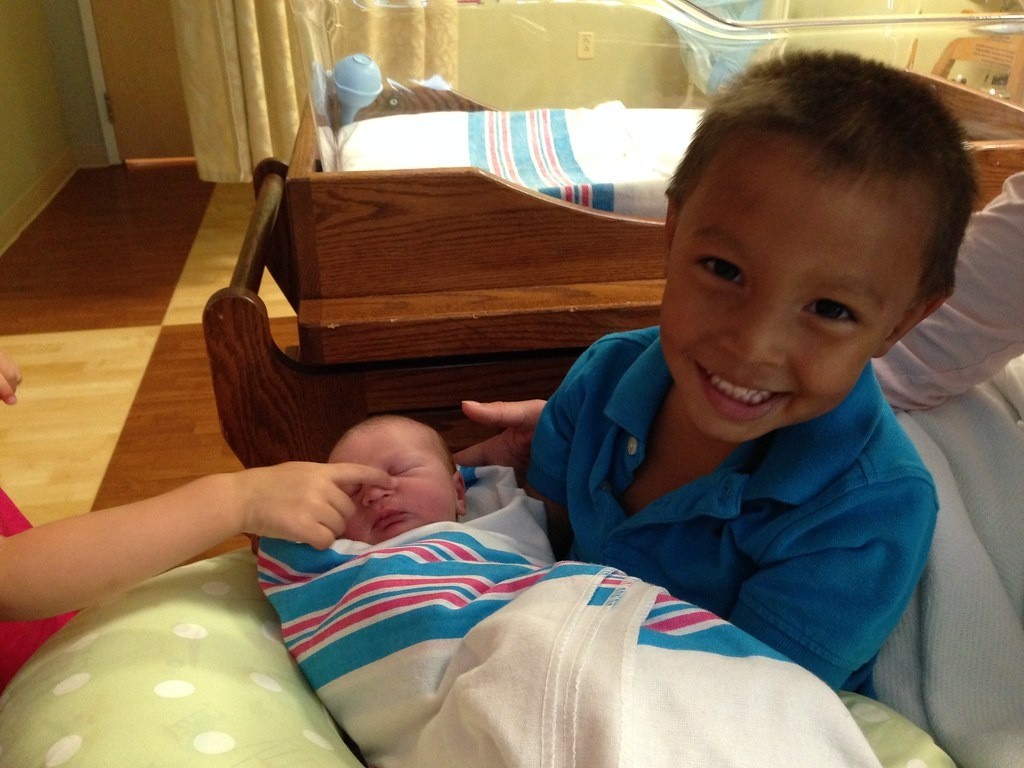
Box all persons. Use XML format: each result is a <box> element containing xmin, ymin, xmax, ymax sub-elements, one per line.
<box><xmin>257</xmin><ymin>415</ymin><xmax>885</xmax><ymax>768</ymax></box>
<box><xmin>524</xmin><ymin>50</ymin><xmax>980</xmax><ymax>696</ymax></box>
<box><xmin>434</xmin><ymin>172</ymin><xmax>1024</xmax><ymax>472</ymax></box>
<box><xmin>0</xmin><ymin>349</ymin><xmax>397</xmax><ymax>695</ymax></box>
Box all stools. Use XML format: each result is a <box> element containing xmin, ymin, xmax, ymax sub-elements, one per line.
<box><xmin>933</xmin><ymin>36</ymin><xmax>1024</xmax><ymax>108</ymax></box>
